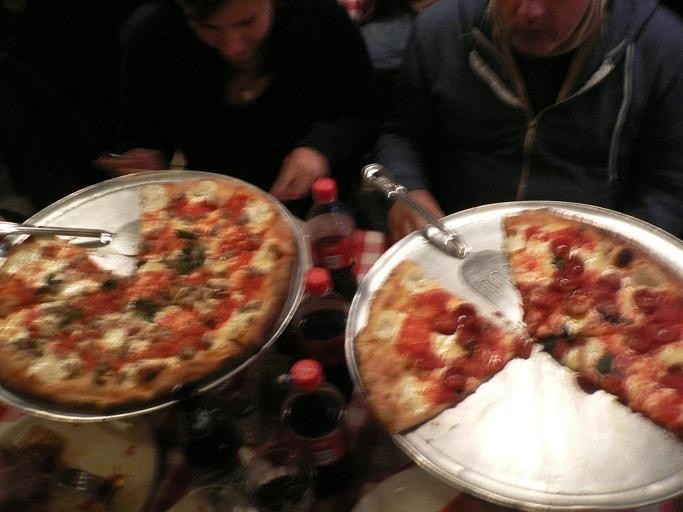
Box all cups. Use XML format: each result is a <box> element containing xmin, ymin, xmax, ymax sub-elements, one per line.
<box><xmin>169</xmin><ymin>448</ymin><xmax>317</xmax><ymax>512</ymax></box>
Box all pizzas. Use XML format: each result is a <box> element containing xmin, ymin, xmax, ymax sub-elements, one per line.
<box><xmin>354</xmin><ymin>209</ymin><xmax>683</xmax><ymax>443</ymax></box>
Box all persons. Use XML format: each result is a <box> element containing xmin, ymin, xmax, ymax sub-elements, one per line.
<box><xmin>357</xmin><ymin>1</ymin><xmax>681</xmax><ymax>249</ymax></box>
<box><xmin>41</xmin><ymin>0</ymin><xmax>379</xmax><ymax>207</ymax></box>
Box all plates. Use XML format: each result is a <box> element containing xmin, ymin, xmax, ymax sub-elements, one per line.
<box><xmin>342</xmin><ymin>198</ymin><xmax>683</xmax><ymax>511</ymax></box>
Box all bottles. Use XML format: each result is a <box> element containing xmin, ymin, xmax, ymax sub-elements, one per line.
<box><xmin>170</xmin><ymin>381</ymin><xmax>231</xmax><ymax>473</ymax></box>
<box><xmin>280</xmin><ymin>177</ymin><xmax>357</xmax><ymax>489</ymax></box>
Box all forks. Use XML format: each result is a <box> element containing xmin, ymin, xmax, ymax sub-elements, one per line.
<box><xmin>48</xmin><ymin>465</ymin><xmax>115</xmax><ymax>503</ymax></box>
